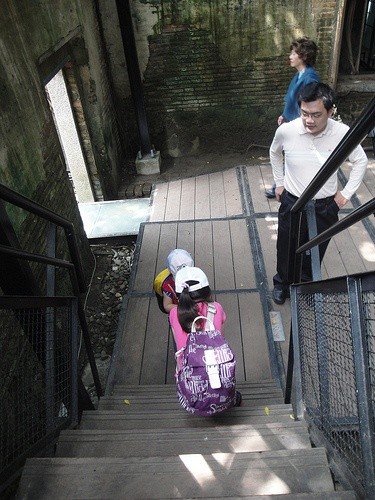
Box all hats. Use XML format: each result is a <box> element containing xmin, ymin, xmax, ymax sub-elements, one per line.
<box><xmin>174</xmin><ymin>266</ymin><xmax>210</xmax><ymax>293</ymax></box>
<box><xmin>168</xmin><ymin>249</ymin><xmax>195</xmax><ymax>278</ymax></box>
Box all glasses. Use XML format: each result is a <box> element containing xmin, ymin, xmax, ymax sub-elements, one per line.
<box><xmin>299</xmin><ymin>111</ymin><xmax>328</xmax><ymax>118</ymax></box>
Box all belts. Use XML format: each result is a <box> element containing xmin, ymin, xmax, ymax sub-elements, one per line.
<box><xmin>285</xmin><ymin>191</ymin><xmax>334</xmax><ymax>203</ymax></box>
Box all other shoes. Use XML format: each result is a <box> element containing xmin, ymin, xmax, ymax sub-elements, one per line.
<box><xmin>265</xmin><ymin>189</ymin><xmax>276</xmax><ymax>198</ymax></box>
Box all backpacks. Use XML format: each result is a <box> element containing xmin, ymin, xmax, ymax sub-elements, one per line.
<box><xmin>175</xmin><ymin>302</ymin><xmax>235</xmax><ymax>417</ymax></box>
<box><xmin>153</xmin><ymin>268</ymin><xmax>180</xmax><ymax>314</ymax></box>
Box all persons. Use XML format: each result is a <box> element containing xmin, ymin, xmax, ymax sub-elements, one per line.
<box><xmin>269</xmin><ymin>80</ymin><xmax>369</xmax><ymax>305</ymax></box>
<box><xmin>265</xmin><ymin>37</ymin><xmax>322</xmax><ymax>197</ymax></box>
<box><xmin>168</xmin><ymin>267</ymin><xmax>241</xmax><ymax>410</ymax></box>
<box><xmin>162</xmin><ymin>248</ymin><xmax>195</xmax><ymax>314</ymax></box>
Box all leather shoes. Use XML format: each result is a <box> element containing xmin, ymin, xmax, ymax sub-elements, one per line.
<box><xmin>273</xmin><ymin>283</ymin><xmax>287</xmax><ymax>304</ymax></box>
